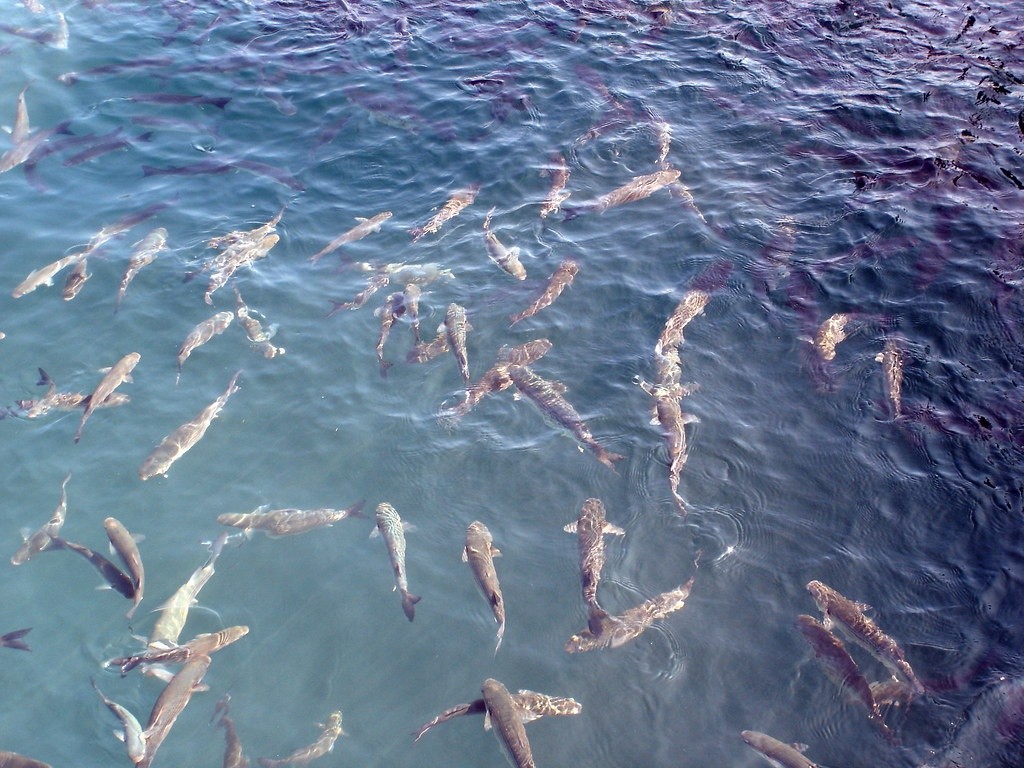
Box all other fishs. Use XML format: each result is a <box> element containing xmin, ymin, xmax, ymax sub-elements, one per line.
<box><xmin>0</xmin><ymin>0</ymin><xmax>932</xmax><ymax>767</ymax></box>
